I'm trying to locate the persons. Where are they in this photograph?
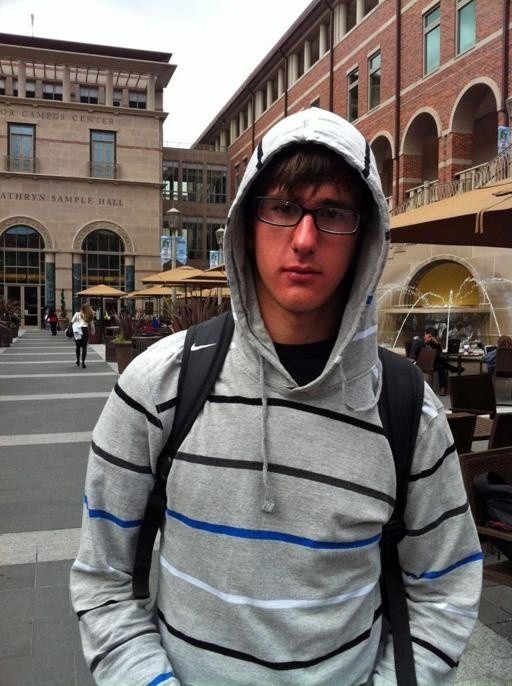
[71,304,96,368]
[46,309,59,336]
[69,106,483,686]
[482,336,512,374]
[410,326,464,396]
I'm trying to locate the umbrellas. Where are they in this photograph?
[76,263,230,318]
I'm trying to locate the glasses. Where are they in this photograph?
[248,192,365,235]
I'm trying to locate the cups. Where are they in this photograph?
[463,344,469,355]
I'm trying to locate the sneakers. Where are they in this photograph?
[75,361,80,367]
[439,387,447,397]
[81,362,88,368]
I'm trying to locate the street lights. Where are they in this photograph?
[209,223,224,316]
[160,207,188,335]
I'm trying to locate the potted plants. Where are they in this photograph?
[57,288,69,331]
[0,296,26,347]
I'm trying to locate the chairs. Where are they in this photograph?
[403,333,511,566]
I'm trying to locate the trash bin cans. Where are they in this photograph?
[131,337,162,360]
[107,328,120,361]
[58,319,69,331]
[89,322,102,344]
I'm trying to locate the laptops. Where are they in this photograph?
[442,338,460,354]
[485,345,497,356]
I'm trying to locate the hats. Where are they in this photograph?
[451,367,465,374]
